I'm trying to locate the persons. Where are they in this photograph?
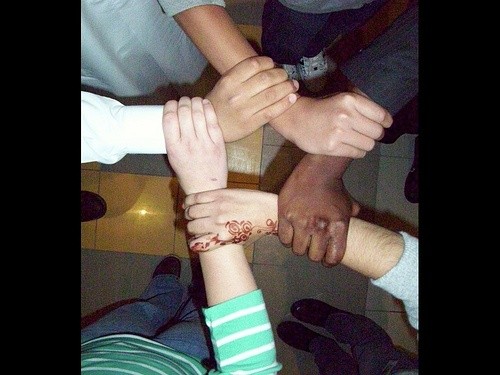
[181,187,418,375]
[279,1,418,267]
[81,55,299,167]
[81,95,285,375]
[81,0,394,159]
[81,189,107,223]
[260,0,390,98]
